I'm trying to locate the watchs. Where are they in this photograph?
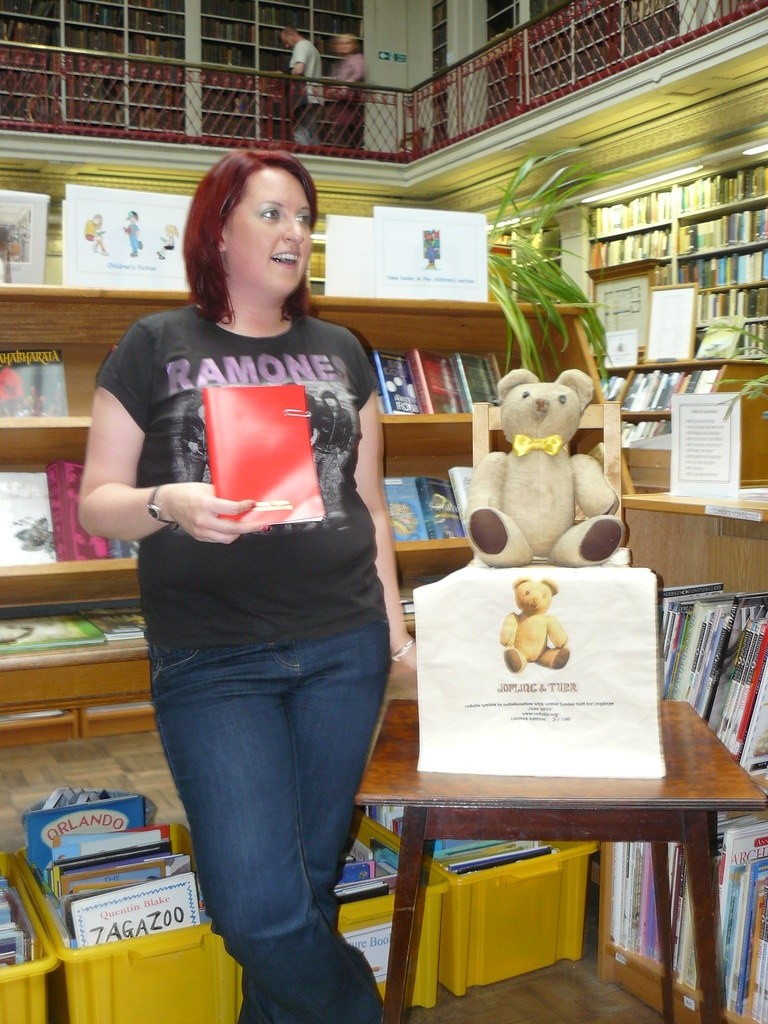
[147,485,176,524]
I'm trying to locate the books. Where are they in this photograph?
[0,0,678,149]
[0,163,768,1024]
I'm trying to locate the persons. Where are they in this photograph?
[79,149,415,1024]
[321,32,367,147]
[277,23,323,149]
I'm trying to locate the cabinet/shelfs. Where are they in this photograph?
[0,0,768,1024]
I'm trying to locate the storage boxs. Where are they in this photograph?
[14,821,238,1024]
[238,816,449,1017]
[0,851,62,1024]
[358,810,599,995]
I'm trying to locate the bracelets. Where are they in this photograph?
[391,638,414,663]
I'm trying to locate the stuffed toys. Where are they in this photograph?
[466,371,625,568]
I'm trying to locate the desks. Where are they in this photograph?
[354,699,768,1024]
